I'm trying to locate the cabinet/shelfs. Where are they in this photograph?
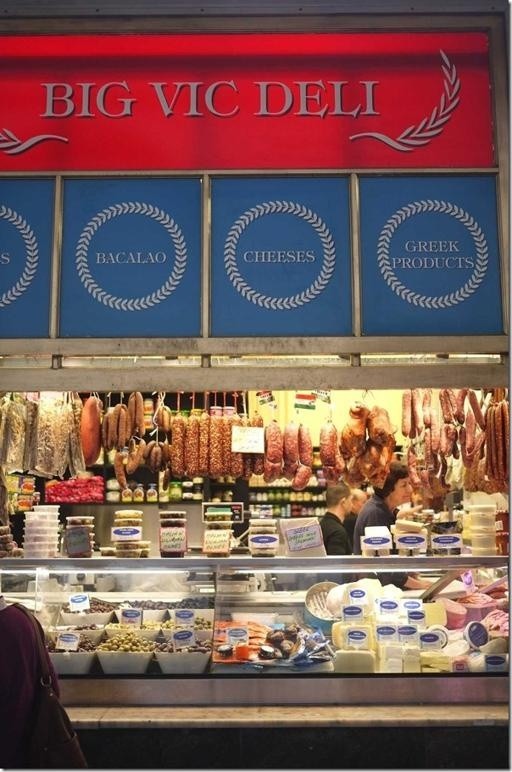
[237,445,402,546]
[64,393,244,501]
[212,557,509,706]
[1,558,211,707]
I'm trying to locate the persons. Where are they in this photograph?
[354,462,413,555]
[319,482,354,556]
[0,595,90,768]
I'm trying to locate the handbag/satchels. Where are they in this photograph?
[10,601,87,769]
